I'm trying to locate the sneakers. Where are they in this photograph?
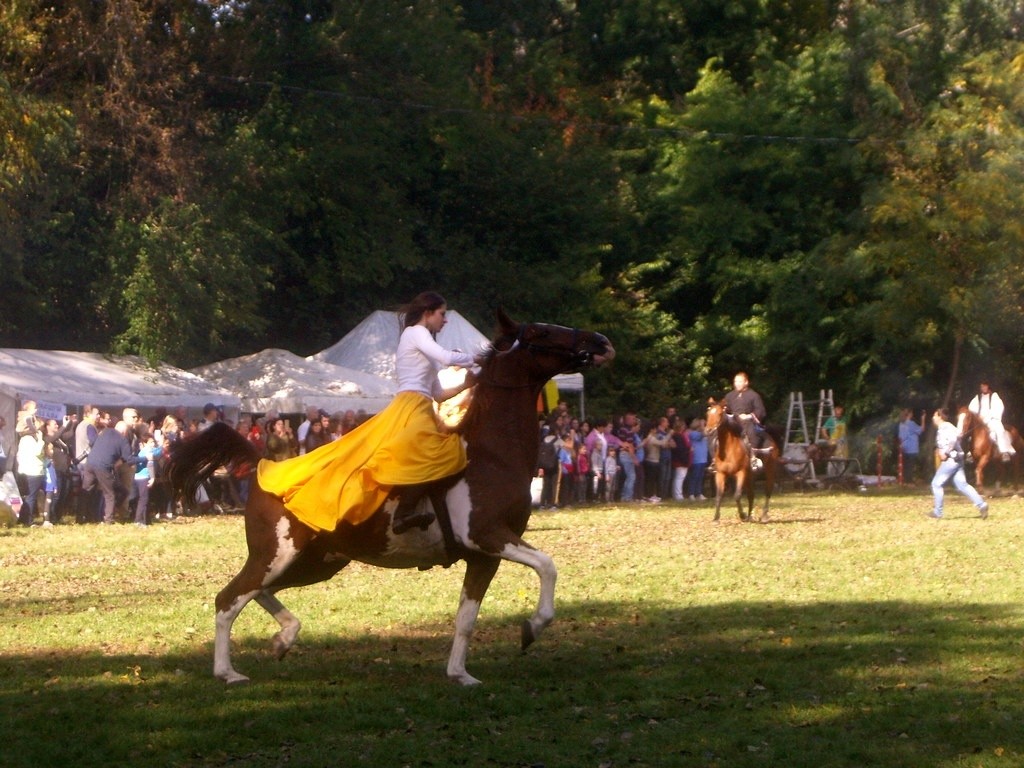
[980,504,989,522]
[926,510,943,519]
[392,502,436,535]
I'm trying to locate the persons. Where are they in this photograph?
[925,408,989,521]
[822,407,849,477]
[723,372,767,471]
[898,408,927,488]
[258,290,481,534]
[0,400,715,528]
[968,379,1016,462]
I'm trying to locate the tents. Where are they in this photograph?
[0,310,585,428]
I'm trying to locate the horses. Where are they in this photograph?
[147,308,617,688]
[954,404,1024,499]
[703,396,780,524]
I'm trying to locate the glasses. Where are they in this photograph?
[132,416,138,419]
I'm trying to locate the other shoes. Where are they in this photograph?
[648,495,662,503]
[698,494,707,500]
[689,495,696,500]
